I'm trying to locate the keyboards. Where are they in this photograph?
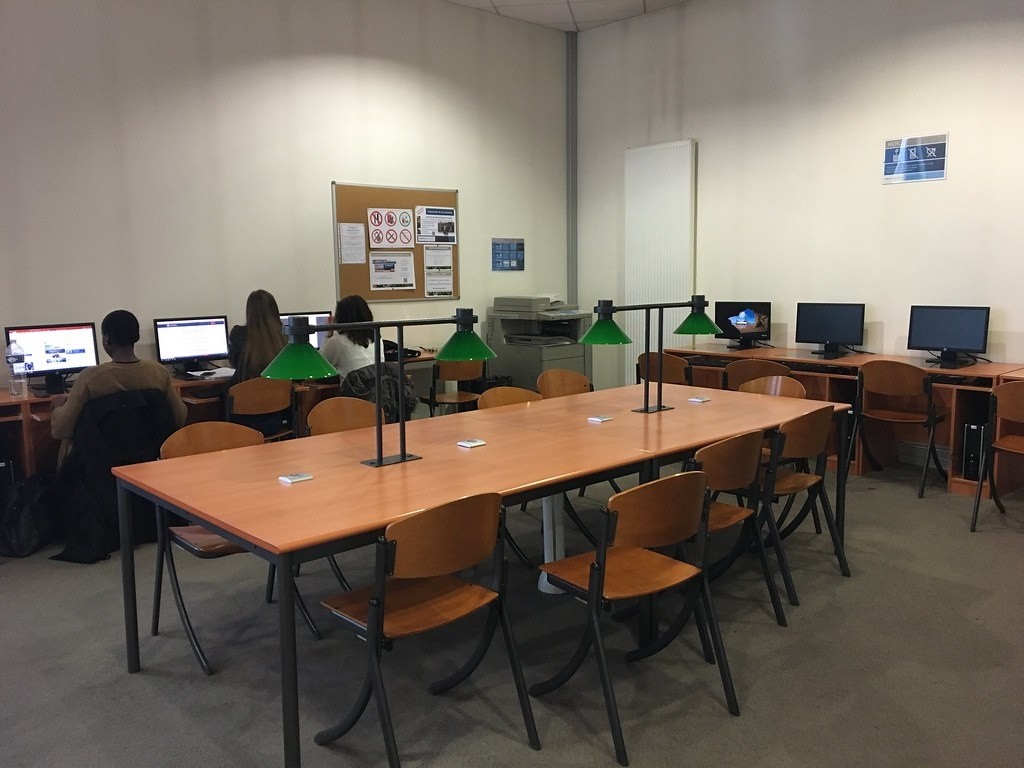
[192,388,226,399]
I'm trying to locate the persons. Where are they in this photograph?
[227,290,289,436]
[319,295,384,387]
[50,310,188,471]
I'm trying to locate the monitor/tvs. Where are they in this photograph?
[153,315,230,379]
[715,302,771,350]
[280,311,332,350]
[796,303,865,359]
[5,322,100,397]
[907,305,990,368]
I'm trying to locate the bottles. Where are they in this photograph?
[4,340,28,401]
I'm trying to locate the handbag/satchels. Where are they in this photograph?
[0,471,65,559]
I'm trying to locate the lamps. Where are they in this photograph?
[260,307,499,468]
[576,294,724,414]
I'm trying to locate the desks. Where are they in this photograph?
[0,350,459,477]
[454,382,853,646]
[662,343,1024,499]
[111,412,653,768]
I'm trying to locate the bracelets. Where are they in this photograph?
[327,335,332,338]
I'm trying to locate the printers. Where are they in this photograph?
[485,296,593,394]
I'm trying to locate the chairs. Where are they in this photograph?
[50,352,1024,768]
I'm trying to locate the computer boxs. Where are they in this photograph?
[0,457,15,510]
[839,397,856,462]
[962,423,992,483]
